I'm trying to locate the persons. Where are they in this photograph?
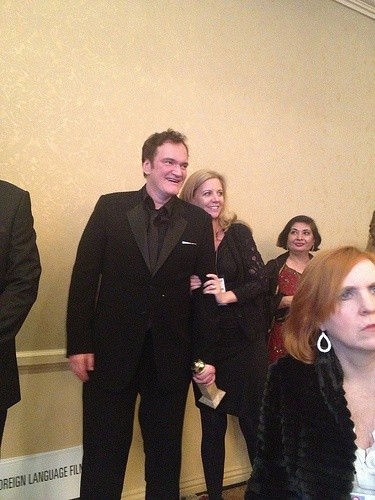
[243,246,375,500]
[66,129,219,500]
[178,171,271,500]
[265,215,323,367]
[0,181,42,445]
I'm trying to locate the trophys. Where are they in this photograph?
[190,358,226,410]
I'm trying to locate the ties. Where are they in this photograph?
[145,204,167,272]
